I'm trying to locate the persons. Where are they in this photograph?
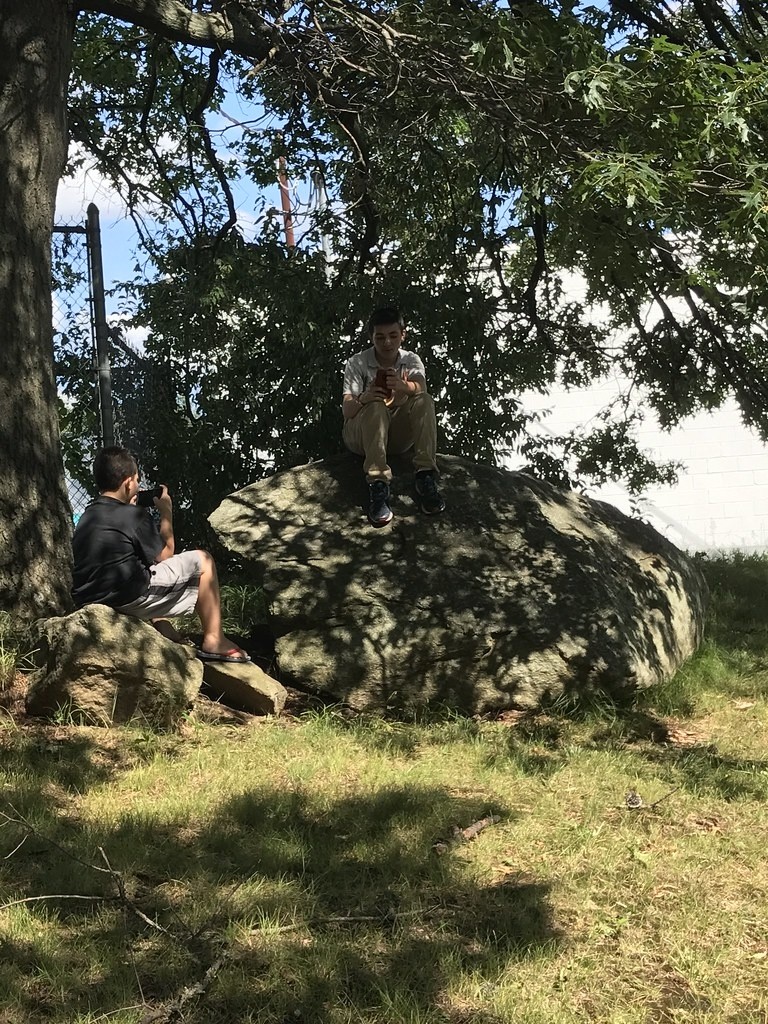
[73,447,252,662]
[342,307,445,524]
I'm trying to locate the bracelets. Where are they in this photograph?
[407,381,417,396]
[356,392,366,407]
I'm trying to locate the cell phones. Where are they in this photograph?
[137,488,163,508]
[375,370,392,399]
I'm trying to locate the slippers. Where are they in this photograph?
[176,636,198,647]
[197,647,249,663]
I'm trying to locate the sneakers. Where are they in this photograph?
[369,480,393,523]
[414,472,445,515]
